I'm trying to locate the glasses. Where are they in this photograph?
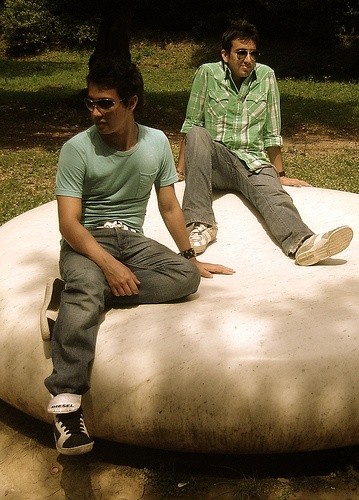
[230,50,260,59]
[84,97,125,112]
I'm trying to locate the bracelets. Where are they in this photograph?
[176,169,183,176]
[279,171,285,176]
[178,249,195,258]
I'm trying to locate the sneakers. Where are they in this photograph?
[294,225,354,266]
[47,393,94,455]
[188,224,218,254]
[40,276,66,342]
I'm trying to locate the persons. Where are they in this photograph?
[39,59,236,457]
[174,19,355,269]
